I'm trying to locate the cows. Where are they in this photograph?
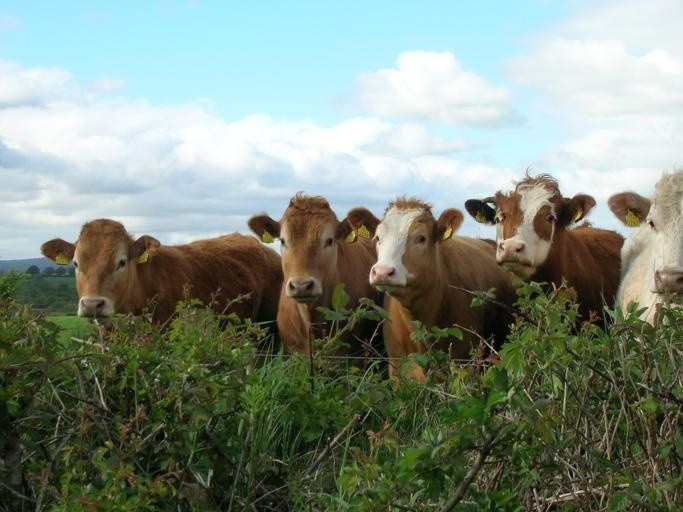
[607,167,682,349]
[464,166,626,341]
[40,219,283,358]
[247,191,384,378]
[368,195,530,396]
